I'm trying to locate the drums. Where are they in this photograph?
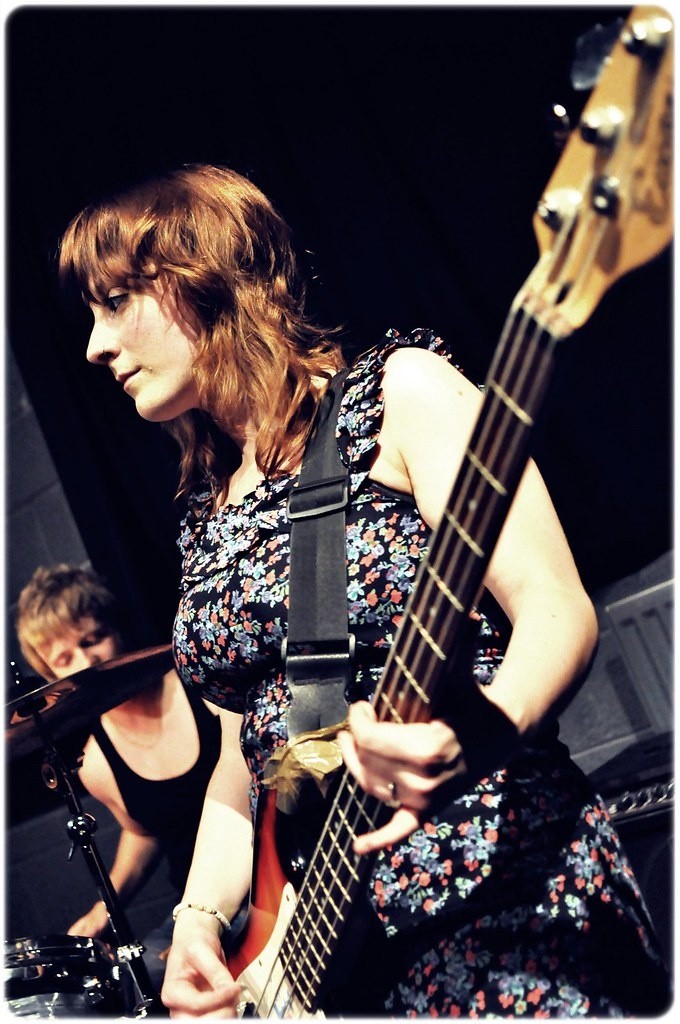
[5,929,146,1019]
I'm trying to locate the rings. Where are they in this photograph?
[385,782,400,808]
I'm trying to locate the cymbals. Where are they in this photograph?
[6,641,172,736]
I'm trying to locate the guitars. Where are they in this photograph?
[230,9,675,1019]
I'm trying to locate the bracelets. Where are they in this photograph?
[172,902,232,932]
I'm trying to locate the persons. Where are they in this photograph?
[17,563,221,938]
[58,163,675,1019]
